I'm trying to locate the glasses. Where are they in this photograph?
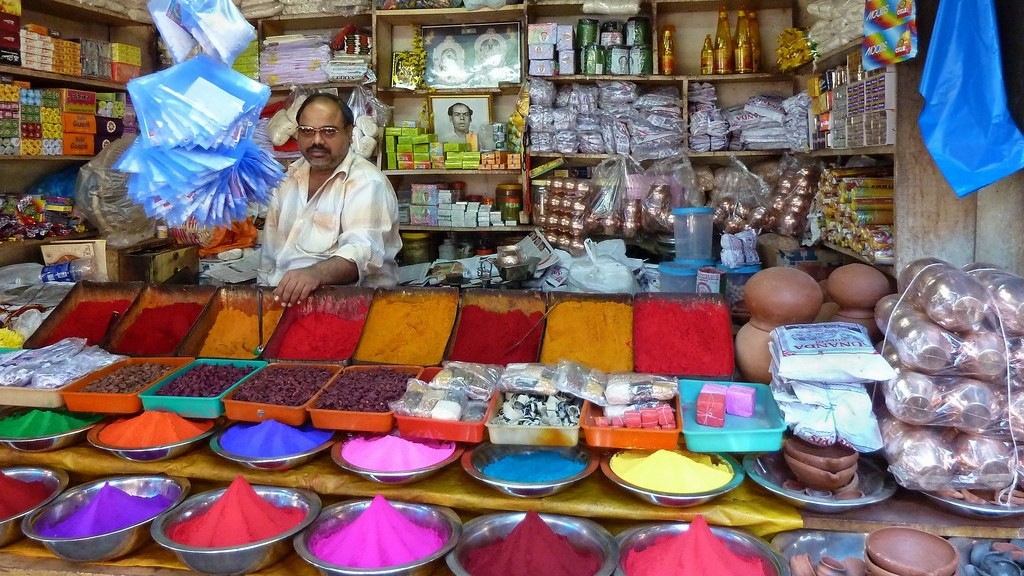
[297,126,347,140]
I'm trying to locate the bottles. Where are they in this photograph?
[401,229,493,265]
[15,258,98,286]
[658,208,760,309]
[661,28,674,77]
[700,9,760,76]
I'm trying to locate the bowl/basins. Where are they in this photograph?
[493,256,541,282]
[0,405,1024,576]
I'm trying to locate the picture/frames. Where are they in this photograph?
[426,93,493,142]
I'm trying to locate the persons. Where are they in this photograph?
[258,93,403,306]
[439,103,473,142]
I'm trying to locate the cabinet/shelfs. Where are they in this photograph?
[0,0,157,245]
[254,0,977,275]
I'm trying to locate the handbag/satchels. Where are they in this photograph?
[567,238,644,296]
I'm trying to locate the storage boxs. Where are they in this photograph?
[111,61,139,85]
[112,43,141,66]
[43,87,96,114]
[0,0,82,76]
[94,115,123,137]
[527,22,574,75]
[385,119,521,228]
[61,132,94,156]
[62,112,96,134]
[93,133,117,155]
